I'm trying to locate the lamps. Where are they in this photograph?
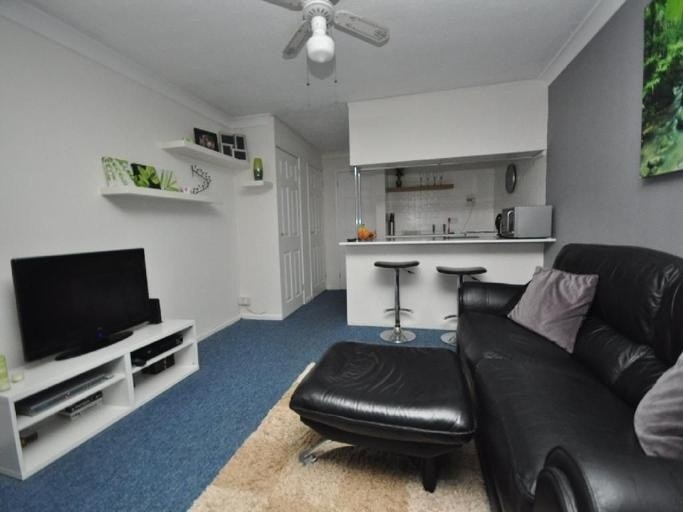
[305,15,335,63]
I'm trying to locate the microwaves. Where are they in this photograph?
[500,205,553,239]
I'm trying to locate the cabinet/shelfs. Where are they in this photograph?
[0,318,199,481]
[274,118,326,322]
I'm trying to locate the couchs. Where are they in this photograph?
[453,243,683,512]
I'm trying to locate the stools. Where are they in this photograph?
[288,339,476,493]
[436,265,486,348]
[375,261,419,344]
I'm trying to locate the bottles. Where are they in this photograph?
[254,157,263,180]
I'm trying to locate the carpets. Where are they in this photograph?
[184,361,491,511]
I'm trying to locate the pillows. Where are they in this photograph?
[506,265,598,356]
[632,349,683,459]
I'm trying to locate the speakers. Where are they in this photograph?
[149,298,161,324]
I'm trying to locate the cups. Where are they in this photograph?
[0,355,10,390]
[344,230,358,242]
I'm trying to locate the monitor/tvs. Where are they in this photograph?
[10,247,151,361]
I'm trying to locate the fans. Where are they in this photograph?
[264,0,388,59]
